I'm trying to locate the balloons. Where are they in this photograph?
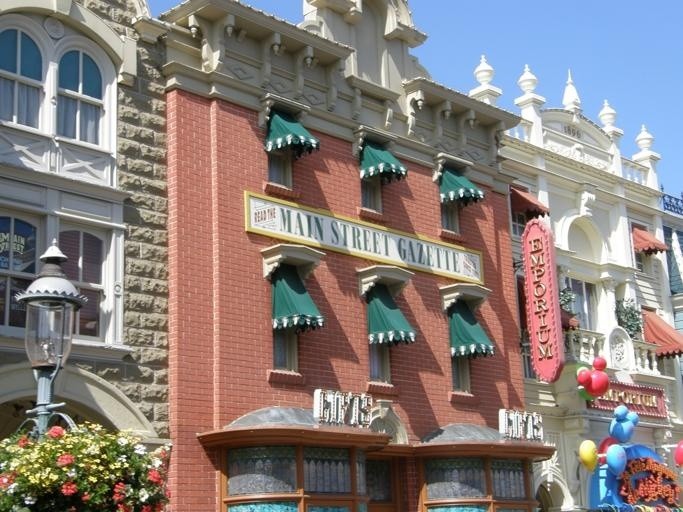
[608,444,627,480]
[674,441,683,465]
[615,405,628,421]
[579,440,597,470]
[574,356,609,401]
[627,412,640,426]
[608,419,634,442]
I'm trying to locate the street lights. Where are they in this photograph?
[15,237,89,442]
